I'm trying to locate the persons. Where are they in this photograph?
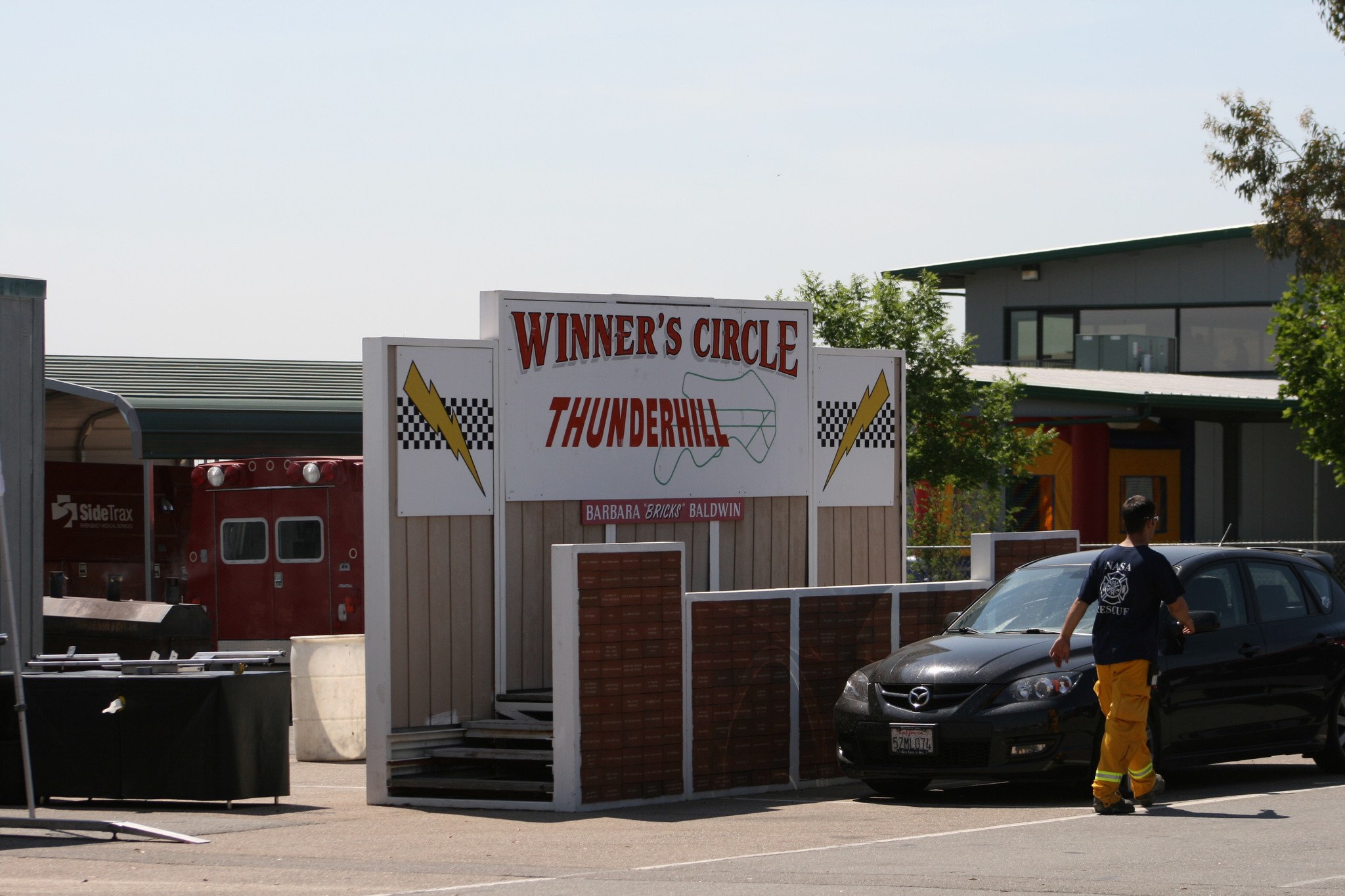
[1048,496,1196,814]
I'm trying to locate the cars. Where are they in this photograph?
[830,547,1345,804]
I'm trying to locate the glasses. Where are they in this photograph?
[1144,515,1159,523]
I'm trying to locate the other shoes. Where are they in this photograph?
[1136,773,1165,807]
[1093,795,1135,814]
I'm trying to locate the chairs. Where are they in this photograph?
[1186,575,1237,626]
[1256,583,1290,619]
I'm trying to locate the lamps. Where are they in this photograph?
[1020,265,1041,282]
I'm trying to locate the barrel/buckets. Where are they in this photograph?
[290,633,364,762]
